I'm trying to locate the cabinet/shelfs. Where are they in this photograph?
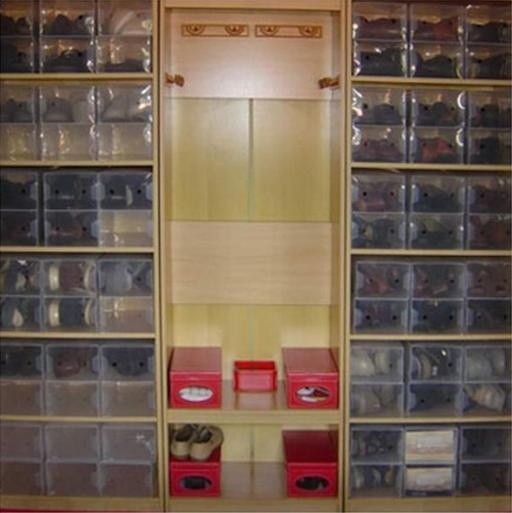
[0,2,161,513]
[162,2,346,512]
[349,1,511,506]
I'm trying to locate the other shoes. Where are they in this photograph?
[351,17,512,493]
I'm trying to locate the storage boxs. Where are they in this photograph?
[349,81,508,174]
[347,165,510,256]
[1,79,152,167]
[349,1,511,87]
[349,421,511,500]
[282,345,341,411]
[3,2,152,81]
[0,250,154,340]
[233,359,277,392]
[2,167,153,253]
[1,419,155,498]
[283,429,338,497]
[349,338,511,420]
[3,336,153,421]
[168,441,222,498]
[167,346,224,409]
[349,255,509,341]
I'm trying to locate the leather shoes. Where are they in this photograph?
[170,424,223,461]
[0,9,152,123]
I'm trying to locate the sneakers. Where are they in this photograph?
[0,174,151,378]
